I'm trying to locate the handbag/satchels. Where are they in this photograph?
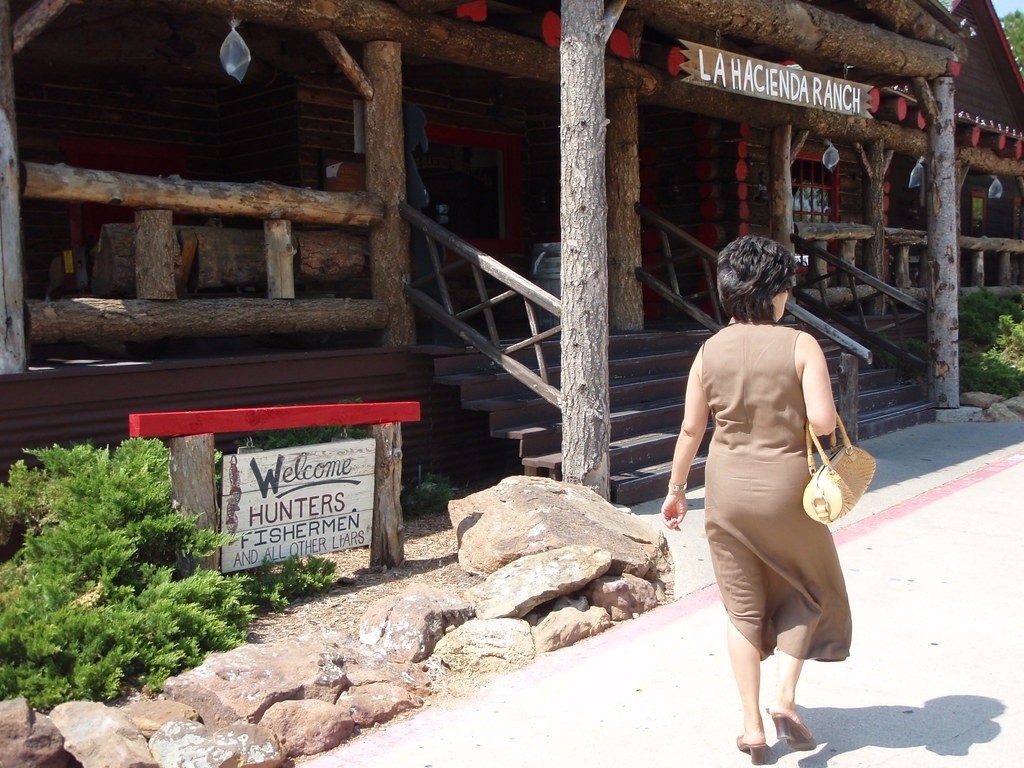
[802,412,876,524]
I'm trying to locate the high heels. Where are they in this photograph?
[771,712,819,752]
[736,734,768,765]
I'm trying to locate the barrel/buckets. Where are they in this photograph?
[529,242,560,340]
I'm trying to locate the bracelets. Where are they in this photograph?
[667,483,687,491]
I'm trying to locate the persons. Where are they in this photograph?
[661,236,853,766]
